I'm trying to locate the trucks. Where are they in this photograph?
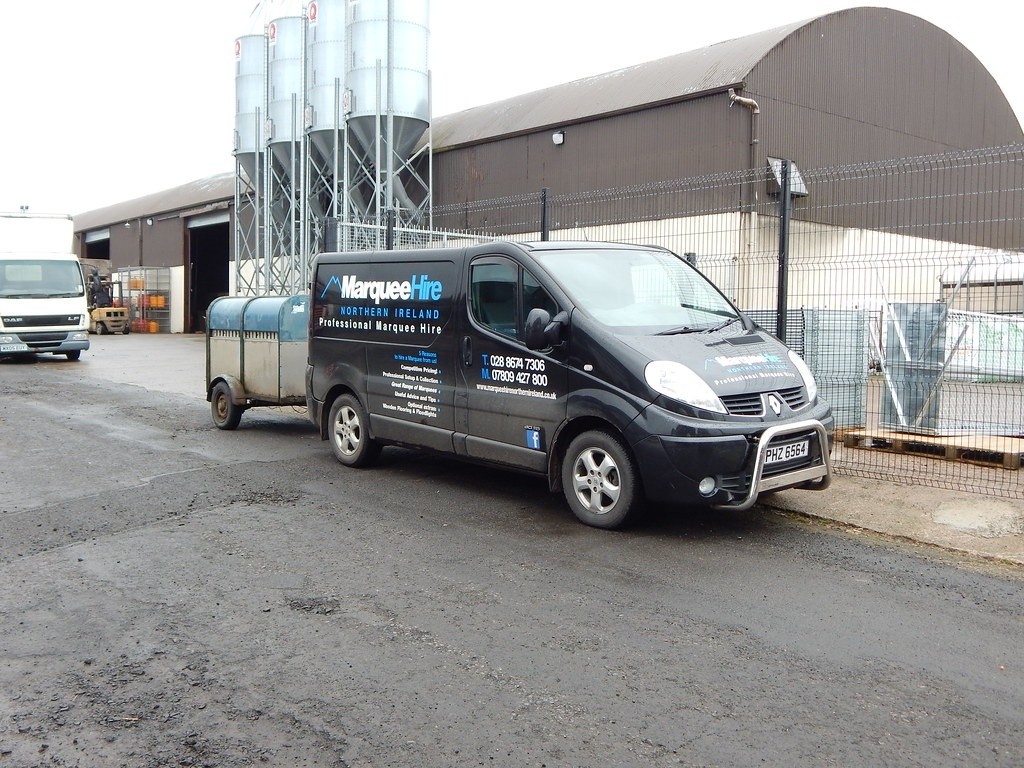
[0,212,90,363]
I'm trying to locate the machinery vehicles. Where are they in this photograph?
[82,274,130,335]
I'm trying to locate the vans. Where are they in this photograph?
[301,240,835,533]
